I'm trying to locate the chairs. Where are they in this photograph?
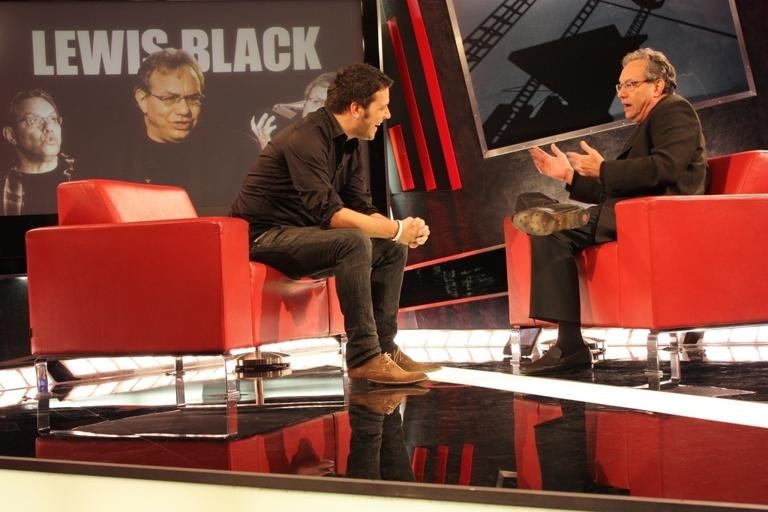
[502,149,767,391]
[24,179,346,442]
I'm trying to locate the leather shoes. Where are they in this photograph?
[386,347,441,373]
[347,351,429,386]
[518,339,595,376]
[510,203,591,237]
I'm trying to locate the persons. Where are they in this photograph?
[228,62,443,386]
[510,49,710,376]
[0,86,77,215]
[250,68,336,151]
[533,365,630,495]
[87,48,260,208]
[287,376,430,481]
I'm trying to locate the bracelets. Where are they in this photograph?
[390,219,402,242]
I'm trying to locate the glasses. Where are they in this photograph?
[144,88,206,107]
[615,77,660,94]
[306,95,325,110]
[14,113,64,129]
